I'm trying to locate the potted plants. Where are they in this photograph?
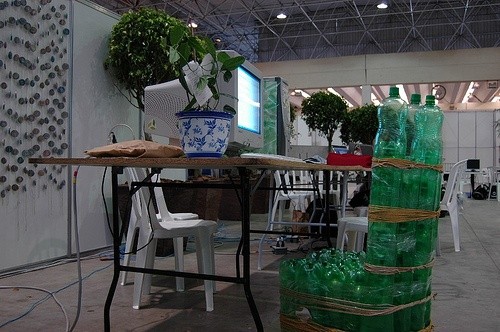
[299,89,350,205]
[156,19,246,156]
[104,9,225,257]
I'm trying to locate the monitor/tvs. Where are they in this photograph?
[144,50,264,156]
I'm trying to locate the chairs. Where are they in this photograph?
[479,167,500,203]
[337,216,372,254]
[280,88,443,332]
[132,167,218,312]
[120,165,198,285]
[434,158,468,255]
[269,156,345,233]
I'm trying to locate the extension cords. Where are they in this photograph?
[272,246,287,255]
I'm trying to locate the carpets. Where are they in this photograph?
[0,209,317,332]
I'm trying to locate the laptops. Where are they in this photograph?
[332,145,348,155]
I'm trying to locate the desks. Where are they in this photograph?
[30,156,372,332]
[444,169,479,198]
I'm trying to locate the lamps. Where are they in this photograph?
[277,9,286,19]
[376,0,388,9]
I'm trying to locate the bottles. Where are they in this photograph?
[410,95,444,166]
[404,94,422,161]
[361,161,441,332]
[373,87,409,159]
[278,248,366,332]
